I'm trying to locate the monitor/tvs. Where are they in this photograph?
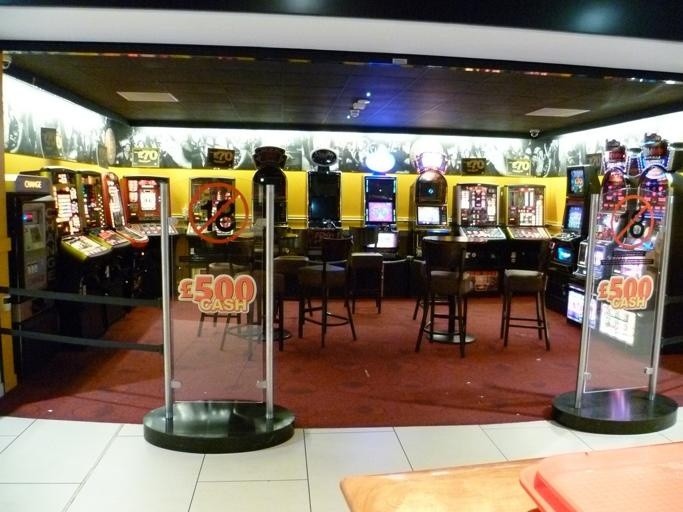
[255,201,287,225]
[459,183,499,226]
[308,196,340,221]
[419,207,441,226]
[554,168,662,278]
[501,185,546,225]
[366,202,394,223]
[47,168,170,234]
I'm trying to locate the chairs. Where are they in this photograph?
[498,239,553,352]
[301,238,357,348]
[413,258,468,335]
[275,235,314,316]
[416,239,476,357]
[343,225,384,314]
[221,241,285,353]
[196,230,254,338]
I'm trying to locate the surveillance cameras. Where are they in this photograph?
[1,54,14,70]
[530,130,541,137]
